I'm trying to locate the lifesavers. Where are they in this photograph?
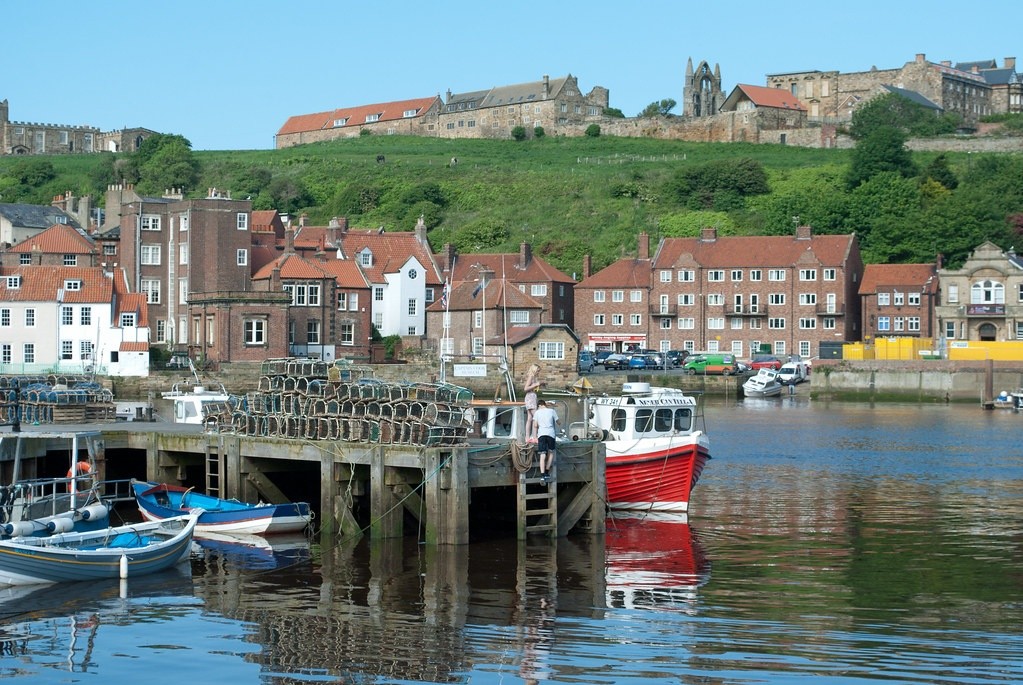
[66,461,98,497]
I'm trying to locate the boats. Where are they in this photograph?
[130,478,313,537]
[1,472,112,540]
[985,389,1023,411]
[440,252,546,444]
[567,376,712,512]
[0,511,201,581]
[741,367,783,396]
[108,381,235,425]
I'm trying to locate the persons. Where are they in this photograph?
[532,400,561,479]
[523,364,544,445]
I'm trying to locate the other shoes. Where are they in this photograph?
[525,438,538,444]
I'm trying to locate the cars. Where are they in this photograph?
[579,348,812,386]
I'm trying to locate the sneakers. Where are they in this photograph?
[545,474,551,479]
[540,476,546,486]
